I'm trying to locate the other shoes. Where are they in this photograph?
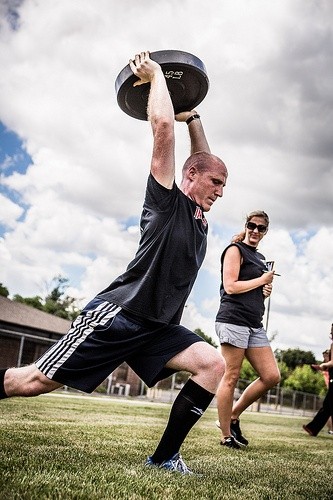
[328,429,333,434]
[303,424,313,437]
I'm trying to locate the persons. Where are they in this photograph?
[0,49,227,478]
[321,350,333,433]
[302,323,333,436]
[215,210,280,449]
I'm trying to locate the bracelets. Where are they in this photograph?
[186,115,200,125]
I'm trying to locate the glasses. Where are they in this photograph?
[246,222,267,233]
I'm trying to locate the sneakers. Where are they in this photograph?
[220,435,247,450]
[216,418,249,447]
[145,453,193,474]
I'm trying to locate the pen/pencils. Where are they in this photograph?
[263,270,282,277]
